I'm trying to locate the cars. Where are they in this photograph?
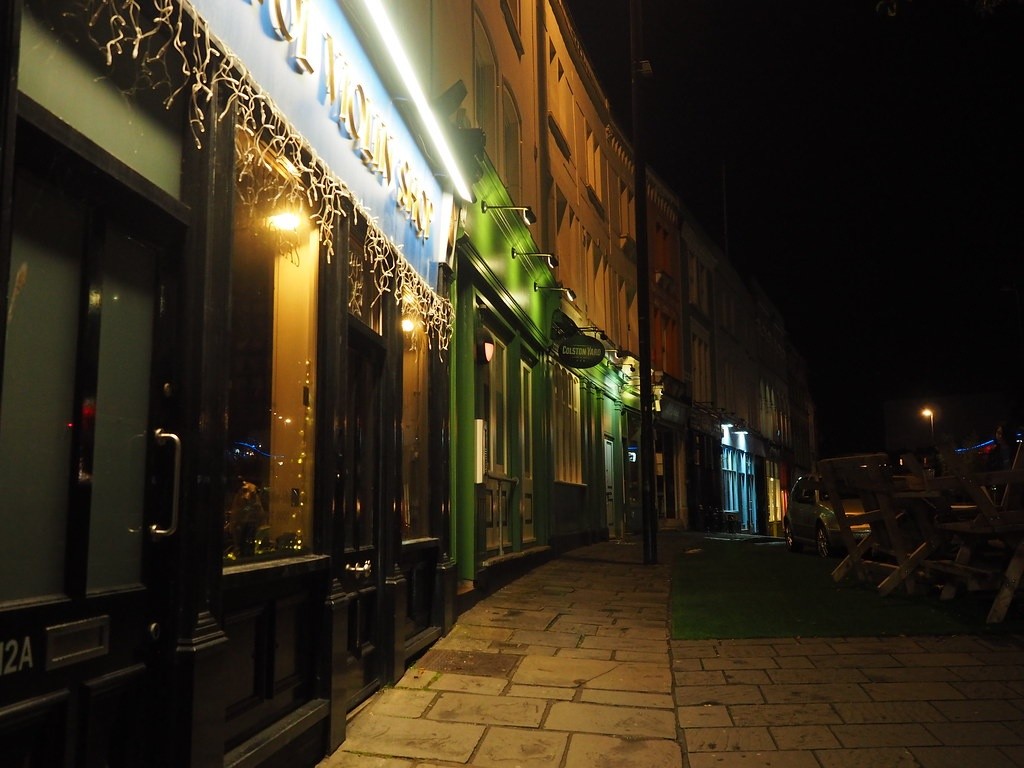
[783,474,888,558]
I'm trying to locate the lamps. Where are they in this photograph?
[400,311,415,331]
[610,363,635,372]
[695,400,746,428]
[582,329,608,340]
[534,281,577,302]
[481,199,537,225]
[605,349,623,359]
[482,329,494,364]
[512,247,559,269]
[256,165,303,232]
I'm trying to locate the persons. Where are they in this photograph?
[985,420,1017,494]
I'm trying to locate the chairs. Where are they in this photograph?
[816,440,1024,626]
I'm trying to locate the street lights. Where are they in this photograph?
[920,406,934,443]
